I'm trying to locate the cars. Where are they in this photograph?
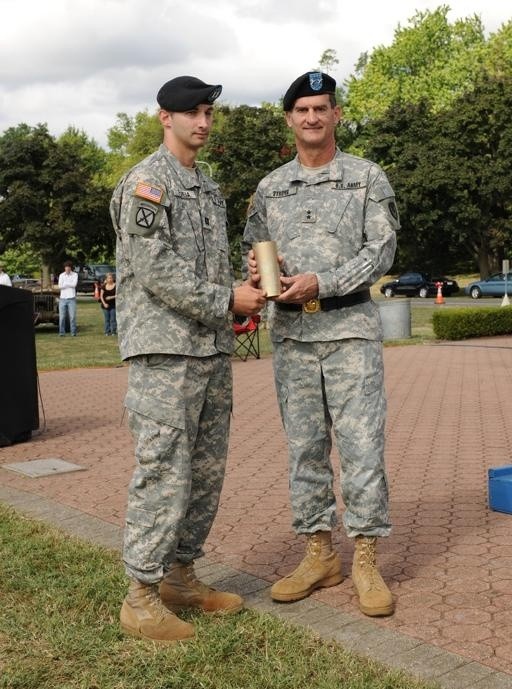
[379,269,463,298]
[14,264,118,295]
[465,271,511,299]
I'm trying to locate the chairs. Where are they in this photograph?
[232,315,261,362]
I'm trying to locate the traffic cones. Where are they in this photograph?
[433,285,446,305]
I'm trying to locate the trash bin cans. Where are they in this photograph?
[0,285,40,448]
[379,301,412,340]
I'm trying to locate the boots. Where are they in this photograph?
[270,531,344,601]
[119,583,196,645]
[160,558,245,617]
[351,535,394,616]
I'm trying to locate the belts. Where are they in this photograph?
[277,290,371,312]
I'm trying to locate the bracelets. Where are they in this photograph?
[229,288,235,311]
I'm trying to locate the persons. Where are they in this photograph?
[100,273,116,337]
[108,75,271,632]
[240,70,401,616]
[58,261,79,336]
[1,266,12,287]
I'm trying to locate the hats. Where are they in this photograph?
[283,71,336,111]
[157,76,222,111]
